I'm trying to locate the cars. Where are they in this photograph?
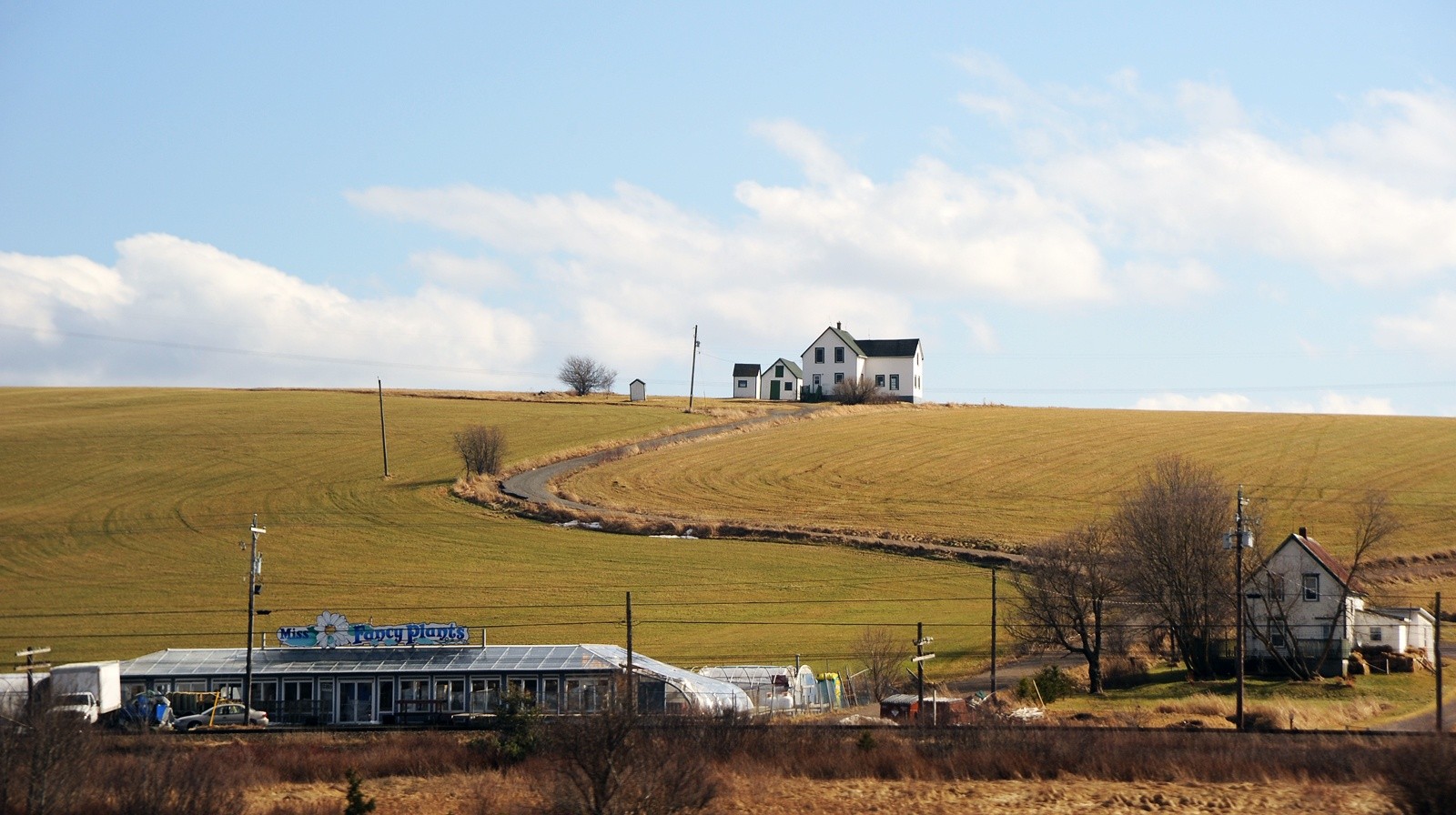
[171,702,271,733]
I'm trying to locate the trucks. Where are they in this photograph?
[43,660,122,725]
